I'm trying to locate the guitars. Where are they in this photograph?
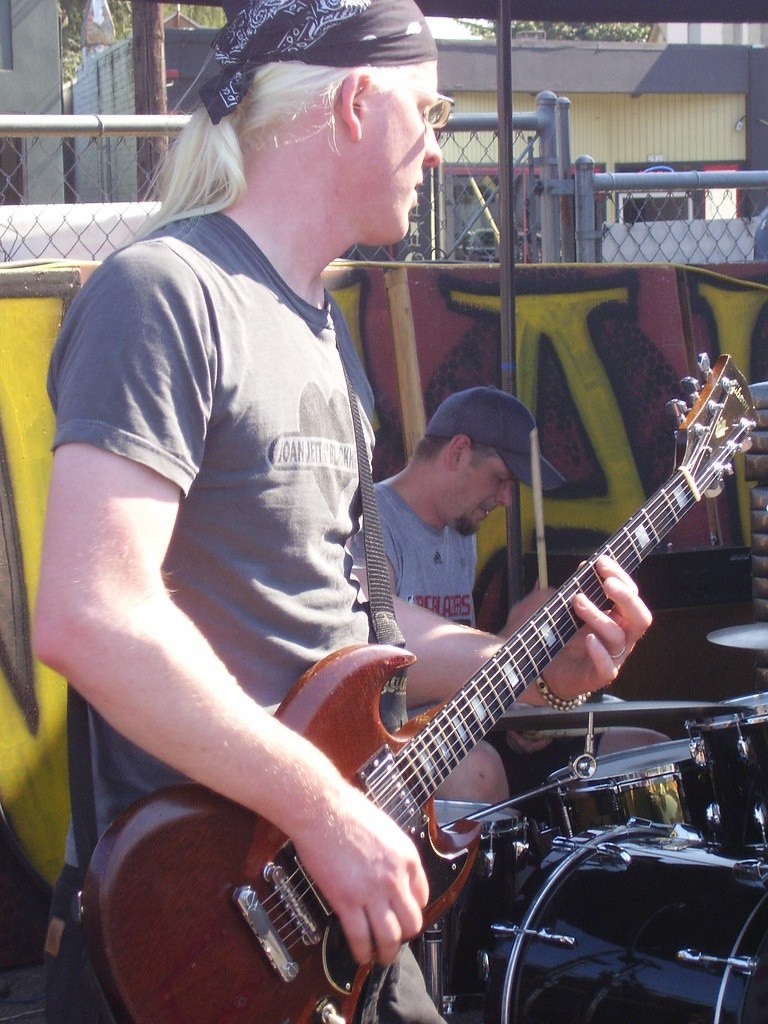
[77,351,763,1024]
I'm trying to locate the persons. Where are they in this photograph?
[350,387,676,830]
[31,0,653,1024]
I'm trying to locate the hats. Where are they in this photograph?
[424,384,567,490]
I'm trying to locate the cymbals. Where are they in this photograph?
[503,698,749,727]
[704,621,767,652]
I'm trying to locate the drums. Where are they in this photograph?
[462,819,768,1024]
[546,735,693,824]
[689,692,768,854]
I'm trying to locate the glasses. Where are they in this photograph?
[335,71,455,129]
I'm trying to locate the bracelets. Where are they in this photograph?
[535,674,592,712]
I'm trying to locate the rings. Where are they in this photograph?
[610,646,625,658]
